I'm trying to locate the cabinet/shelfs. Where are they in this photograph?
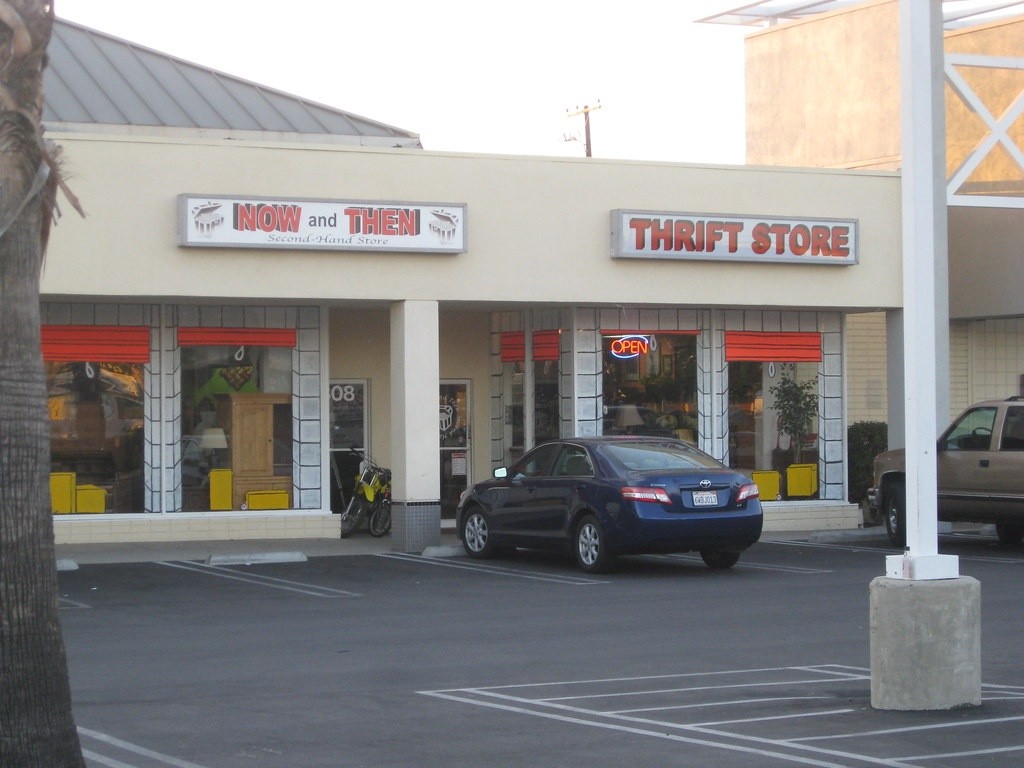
[215,392,292,508]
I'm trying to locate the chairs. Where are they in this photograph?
[566,456,591,475]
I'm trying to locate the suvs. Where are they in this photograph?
[873,396,1024,548]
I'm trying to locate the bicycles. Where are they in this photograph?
[334,447,394,538]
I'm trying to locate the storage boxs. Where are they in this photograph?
[752,469,779,501]
[786,463,817,497]
[206,470,234,511]
[48,470,76,514]
[245,489,292,510]
[74,484,106,513]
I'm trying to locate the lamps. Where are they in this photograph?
[199,428,228,467]
[612,404,646,436]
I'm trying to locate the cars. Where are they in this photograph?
[456,435,764,577]
